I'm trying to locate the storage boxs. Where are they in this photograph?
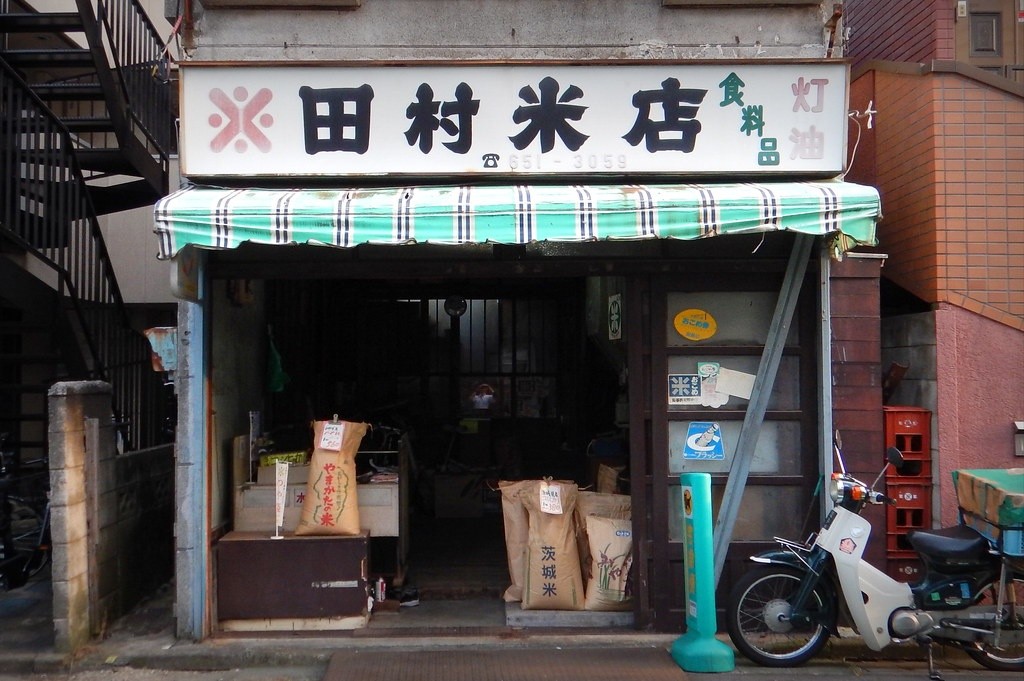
[423,469,483,519]
[883,405,932,482]
[888,558,926,585]
[886,481,933,558]
[952,468,1024,556]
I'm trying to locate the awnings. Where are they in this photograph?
[154,184,883,261]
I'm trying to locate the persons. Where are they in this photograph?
[470,384,497,409]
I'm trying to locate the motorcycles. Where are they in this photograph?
[725,427,1023,681]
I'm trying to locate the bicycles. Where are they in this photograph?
[0,456,52,590]
